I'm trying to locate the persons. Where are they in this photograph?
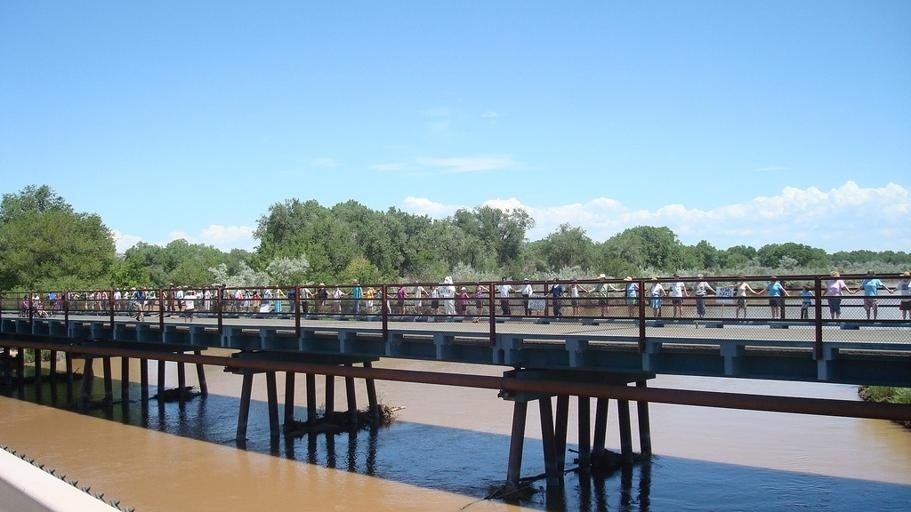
[823,270,853,319]
[162,281,218,317]
[757,275,790,319]
[799,285,815,319]
[113,285,156,322]
[547,274,716,317]
[395,272,534,315]
[728,281,757,318]
[892,272,911,320]
[222,283,295,312]
[23,290,108,317]
[853,269,892,319]
[299,279,393,313]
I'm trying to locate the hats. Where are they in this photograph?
[524,278,529,281]
[673,272,679,277]
[624,276,632,281]
[597,274,606,278]
